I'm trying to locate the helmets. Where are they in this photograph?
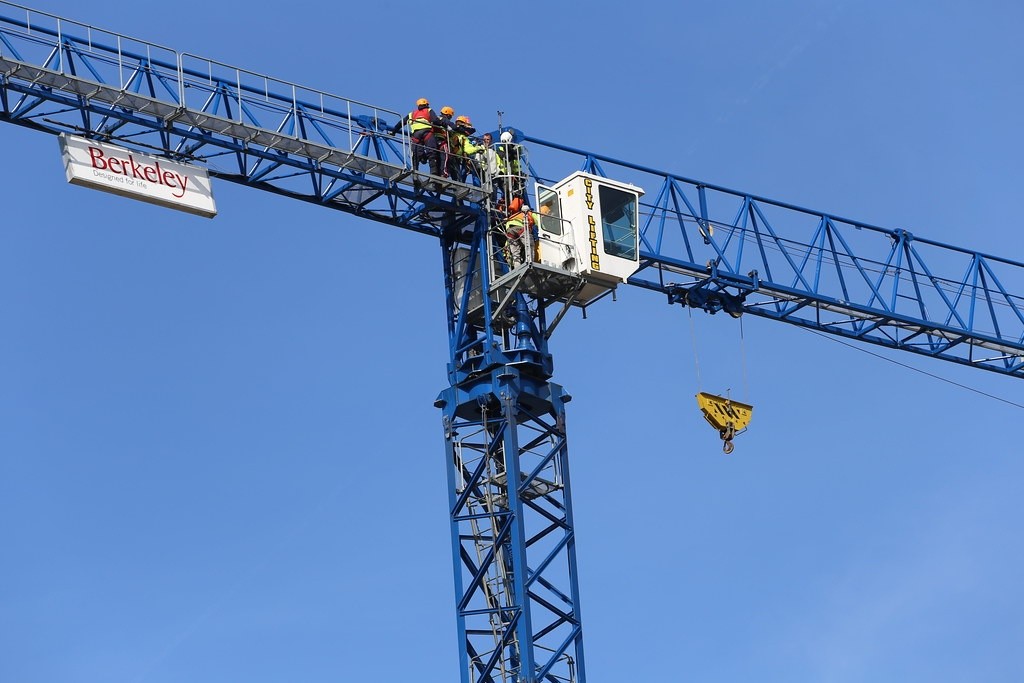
[500,132,512,142]
[541,206,550,217]
[456,116,467,124]
[417,99,429,106]
[440,106,453,117]
[521,205,530,212]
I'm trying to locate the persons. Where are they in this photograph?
[385,98,551,269]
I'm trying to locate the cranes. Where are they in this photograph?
[0,2,1023,682]
